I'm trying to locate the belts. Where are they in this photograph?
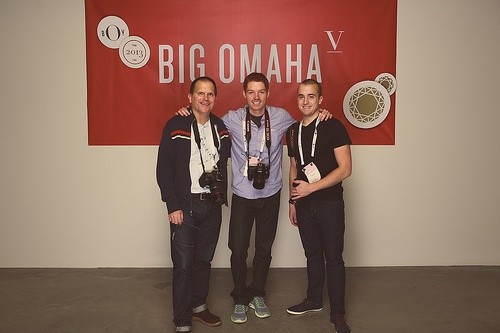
[191,192,211,200]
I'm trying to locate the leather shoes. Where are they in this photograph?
[193,308,221,326]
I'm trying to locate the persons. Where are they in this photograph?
[176,72,332,323]
[157,77,232,333]
[286,79,352,333]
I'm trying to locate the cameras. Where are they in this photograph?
[248,163,269,190]
[288,178,311,207]
[199,169,226,206]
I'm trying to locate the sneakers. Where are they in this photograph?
[331,316,350,333]
[249,296,270,318]
[287,298,322,314]
[231,305,248,323]
